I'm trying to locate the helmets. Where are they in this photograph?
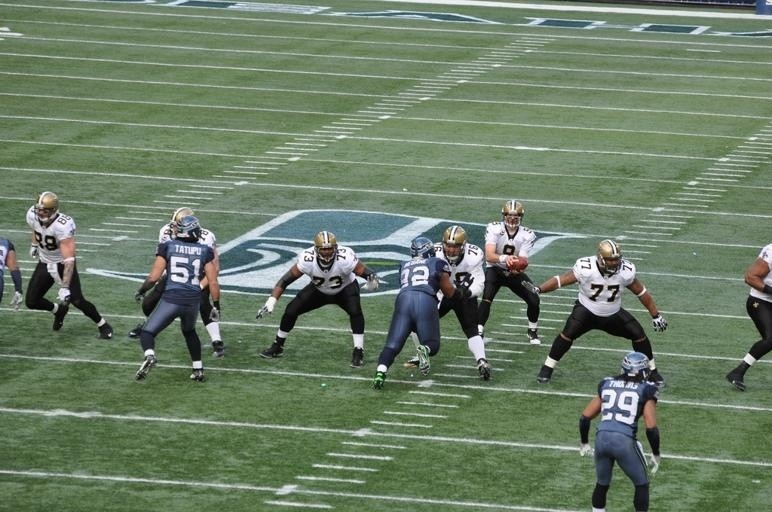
[501,200,525,228]
[169,207,194,232]
[620,352,650,378]
[441,225,468,261]
[410,237,436,260]
[596,239,623,275]
[314,231,337,262]
[173,215,202,240]
[31,191,59,223]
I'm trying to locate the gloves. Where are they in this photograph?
[521,281,541,295]
[579,442,595,457]
[649,453,661,473]
[29,243,39,259]
[10,289,23,311]
[57,287,71,306]
[652,312,668,332]
[256,297,277,319]
[209,300,220,322]
[360,274,379,292]
[134,289,146,304]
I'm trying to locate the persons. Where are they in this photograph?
[525,239,668,386]
[26,192,112,340]
[372,237,456,389]
[478,200,540,345]
[404,225,491,382]
[134,217,220,385]
[726,242,772,390]
[0,238,23,309]
[256,231,379,367]
[580,353,661,511]
[128,207,224,359]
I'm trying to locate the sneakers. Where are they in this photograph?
[536,365,553,385]
[407,356,420,366]
[416,345,431,377]
[368,371,386,390]
[648,367,665,388]
[189,368,205,381]
[53,304,69,331]
[725,368,746,392]
[349,345,364,368]
[526,328,540,345]
[98,323,113,339]
[135,354,158,380]
[477,358,491,380]
[127,321,146,337]
[212,340,225,358]
[259,342,283,359]
